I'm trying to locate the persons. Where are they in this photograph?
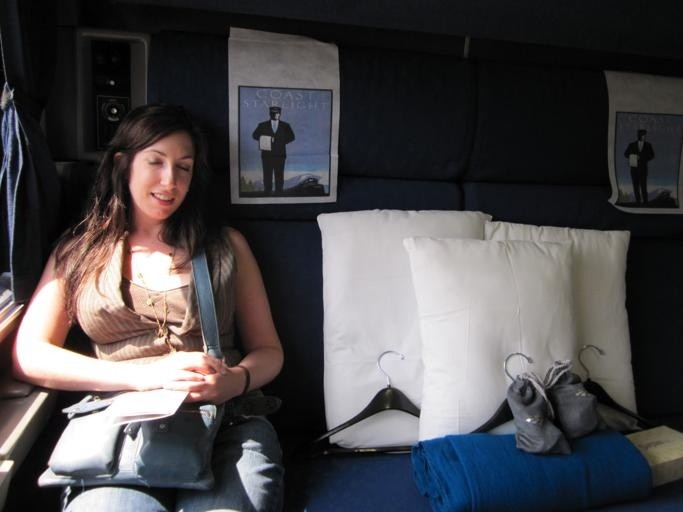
[11,100,286,512]
[624,128,655,202]
[252,105,296,190]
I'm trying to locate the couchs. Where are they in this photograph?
[41,10,683,512]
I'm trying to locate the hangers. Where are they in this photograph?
[470,353,540,436]
[320,351,420,441]
[580,345,639,427]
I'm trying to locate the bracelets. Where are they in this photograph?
[236,362,252,396]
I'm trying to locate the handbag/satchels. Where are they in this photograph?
[37,390,224,492]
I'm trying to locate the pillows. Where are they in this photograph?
[483,224,636,416]
[318,210,490,445]
[405,237,579,441]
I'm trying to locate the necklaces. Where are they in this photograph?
[126,231,179,352]
